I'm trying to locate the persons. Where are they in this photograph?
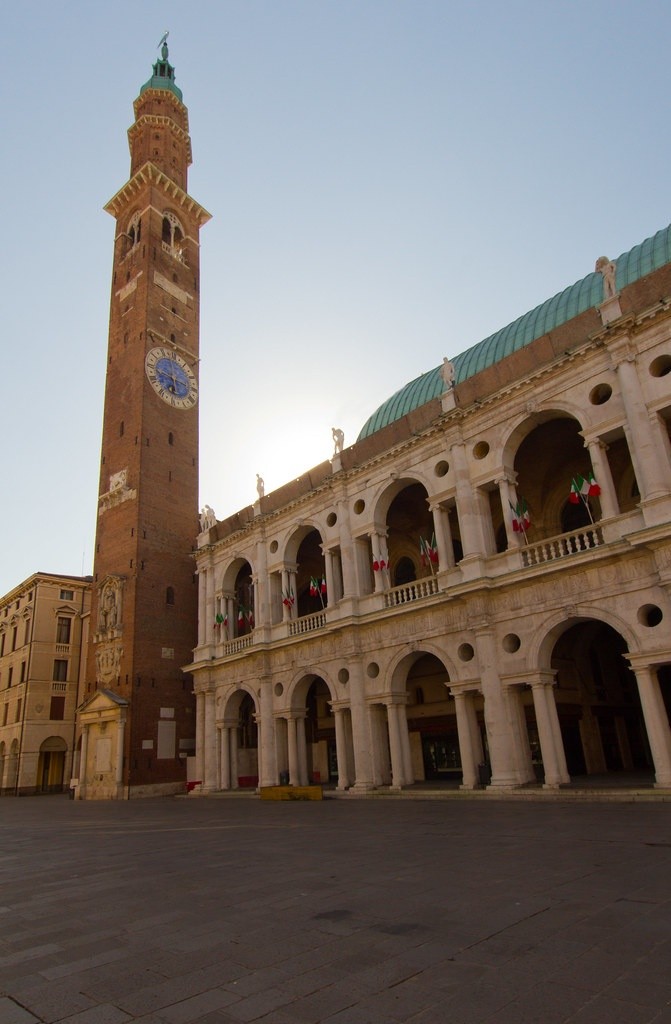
[595,256,616,300]
[256,474,264,498]
[198,504,216,532]
[332,428,344,455]
[440,357,455,389]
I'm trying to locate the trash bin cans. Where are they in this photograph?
[280,771,289,786]
[313,771,321,784]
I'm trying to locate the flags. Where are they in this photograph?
[587,471,601,497]
[372,550,391,572]
[509,502,523,533]
[310,574,326,597]
[420,532,439,567]
[576,473,590,495]
[515,501,522,525]
[281,586,294,610]
[568,478,581,504]
[213,613,228,630]
[237,604,255,630]
[521,496,531,529]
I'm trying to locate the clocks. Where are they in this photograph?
[144,346,198,411]
